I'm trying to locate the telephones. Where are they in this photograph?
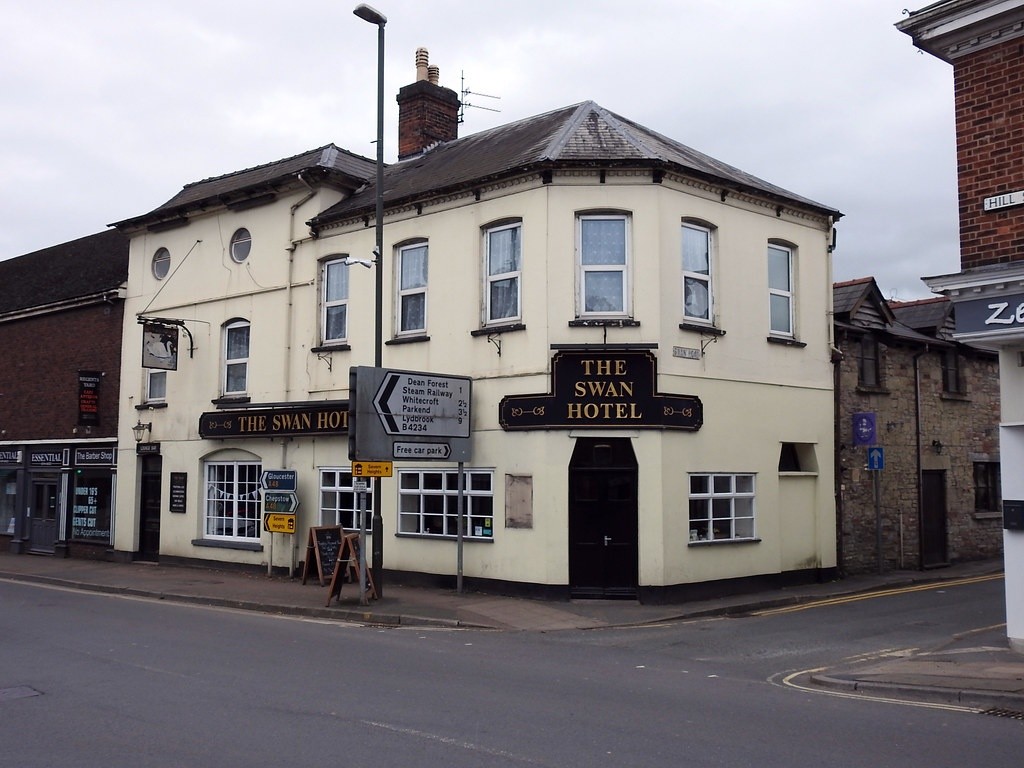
[312,525,344,578]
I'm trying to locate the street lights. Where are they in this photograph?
[352,2,387,600]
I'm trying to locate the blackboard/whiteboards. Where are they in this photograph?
[347,534,375,595]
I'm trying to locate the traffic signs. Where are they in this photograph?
[349,364,474,464]
[262,513,298,534]
[264,491,300,513]
[259,469,298,493]
